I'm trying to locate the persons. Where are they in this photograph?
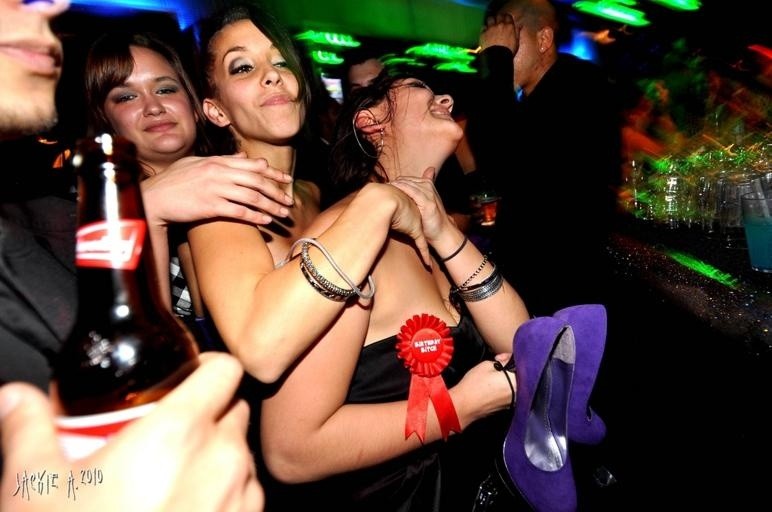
[0,1,626,512]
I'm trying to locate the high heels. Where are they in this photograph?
[503,304,607,510]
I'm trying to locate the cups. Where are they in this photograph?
[743,190,772,273]
[471,192,502,227]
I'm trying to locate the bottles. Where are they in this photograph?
[47,134,200,465]
